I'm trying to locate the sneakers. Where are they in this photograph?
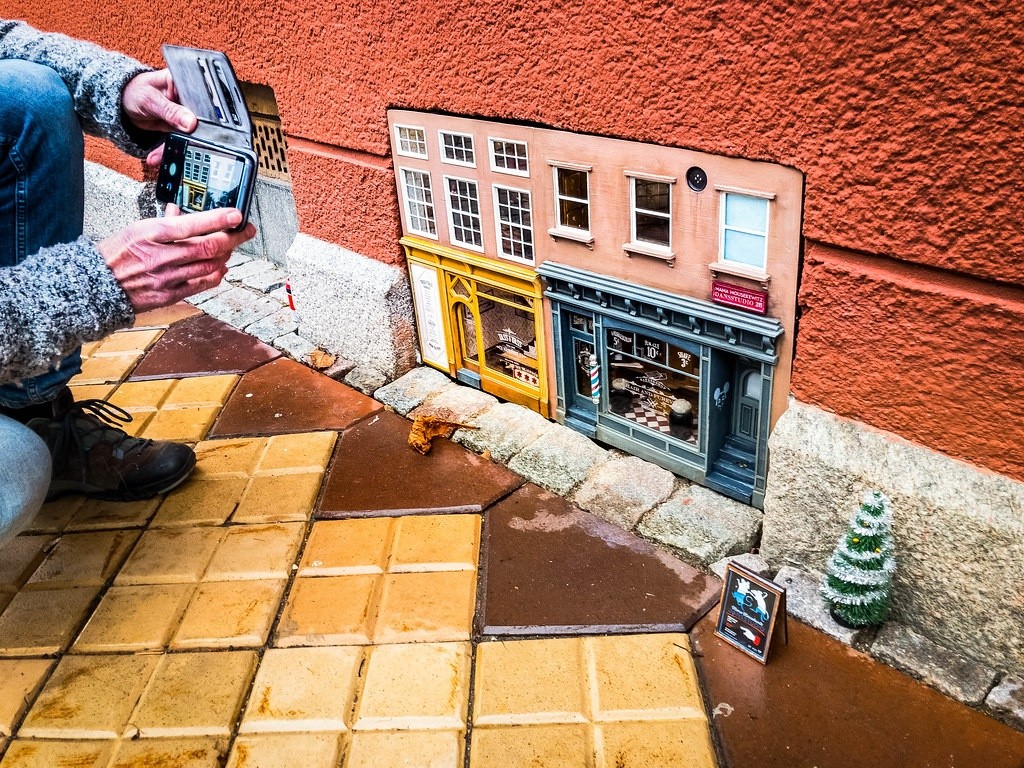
[23,386,197,503]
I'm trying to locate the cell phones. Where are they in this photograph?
[155,133,255,231]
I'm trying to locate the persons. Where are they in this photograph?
[0,17,257,542]
